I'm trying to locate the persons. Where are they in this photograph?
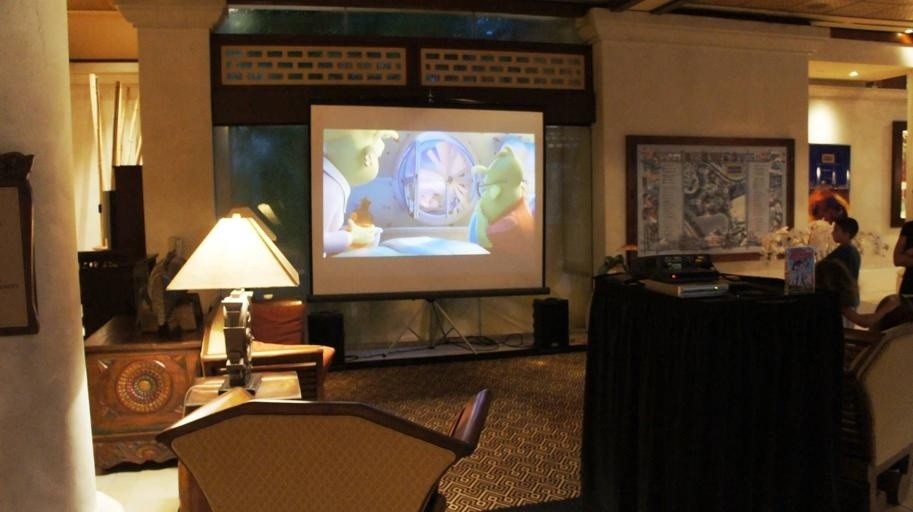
[891,221,912,298]
[816,216,862,329]
[147,253,188,341]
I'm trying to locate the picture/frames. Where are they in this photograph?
[889,121,907,226]
[621,132,798,267]
[0,151,43,336]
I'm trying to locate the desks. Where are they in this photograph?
[184,371,304,413]
[574,270,844,511]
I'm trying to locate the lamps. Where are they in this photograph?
[164,213,301,396]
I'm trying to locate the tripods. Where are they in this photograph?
[381,299,478,357]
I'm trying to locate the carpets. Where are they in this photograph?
[313,349,592,508]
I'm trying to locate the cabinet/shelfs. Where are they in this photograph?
[83,309,206,477]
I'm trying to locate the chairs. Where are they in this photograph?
[843,319,911,506]
[154,385,492,510]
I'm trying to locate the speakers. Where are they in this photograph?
[308,311,345,363]
[533,299,570,344]
